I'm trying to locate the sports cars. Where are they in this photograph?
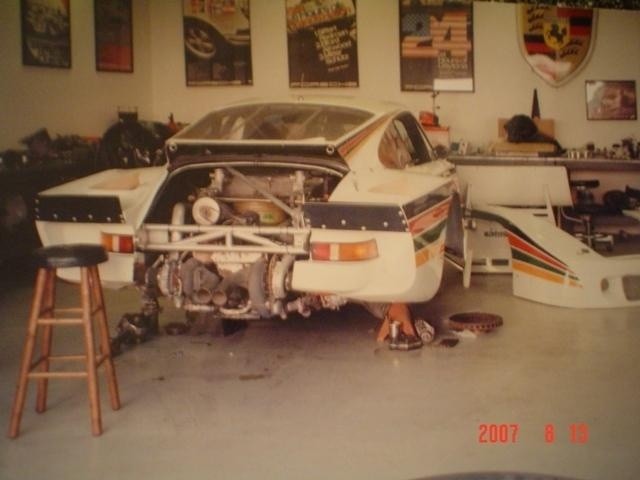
[29,80,480,324]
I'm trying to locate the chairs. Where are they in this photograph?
[564,177,617,255]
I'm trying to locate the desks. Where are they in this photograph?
[448,150,640,206]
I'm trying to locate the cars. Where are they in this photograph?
[184,2,251,62]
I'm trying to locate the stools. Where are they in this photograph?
[4,240,127,441]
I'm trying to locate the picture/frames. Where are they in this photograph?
[583,76,638,125]
